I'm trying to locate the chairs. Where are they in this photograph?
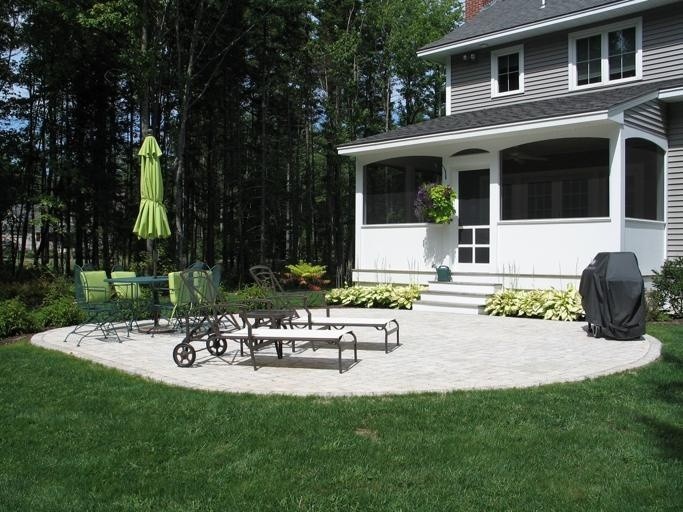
[172,269,357,374]
[64,259,242,347]
[239,264,400,354]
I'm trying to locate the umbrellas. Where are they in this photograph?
[130,128,173,278]
